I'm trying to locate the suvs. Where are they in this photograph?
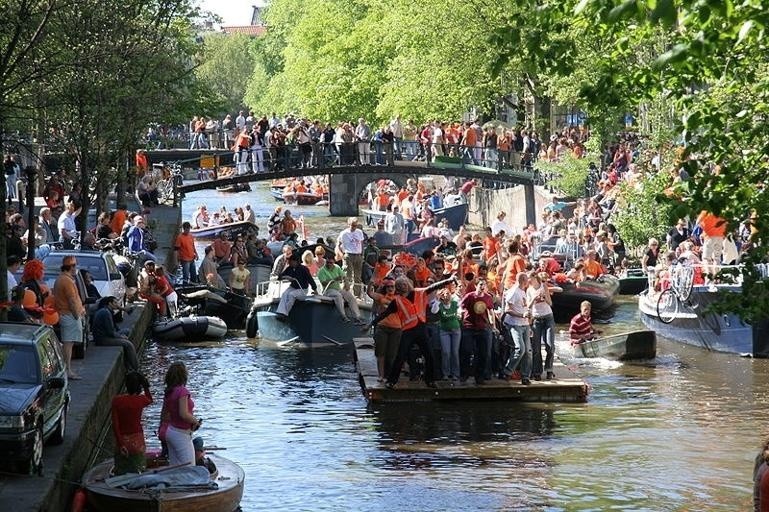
[0,322,72,478]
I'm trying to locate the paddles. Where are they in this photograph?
[104,461,191,488]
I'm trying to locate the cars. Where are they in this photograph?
[13,251,125,352]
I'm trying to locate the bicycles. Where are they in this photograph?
[656,269,697,323]
[157,176,182,205]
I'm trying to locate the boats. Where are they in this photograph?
[152,182,648,348]
[574,329,658,357]
[78,447,245,511]
[349,336,589,405]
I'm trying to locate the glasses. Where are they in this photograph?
[387,285,394,288]
[238,262,245,265]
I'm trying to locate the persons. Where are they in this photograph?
[361,112,603,390]
[751,436,769,512]
[110,370,154,475]
[192,436,210,470]
[159,359,203,468]
[581,111,768,293]
[1,108,374,382]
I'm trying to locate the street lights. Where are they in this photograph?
[22,134,42,260]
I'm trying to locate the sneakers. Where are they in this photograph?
[379,371,554,389]
[340,317,369,326]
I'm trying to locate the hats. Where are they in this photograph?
[183,222,192,228]
[386,280,395,286]
[134,216,144,223]
[63,256,76,266]
